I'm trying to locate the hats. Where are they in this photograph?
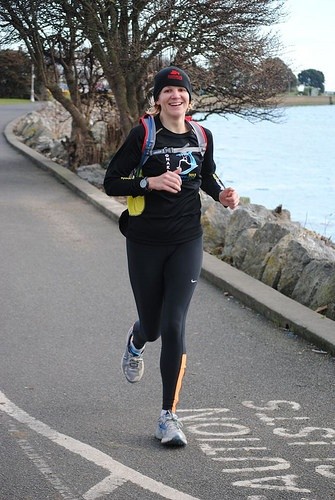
[153,66,191,104]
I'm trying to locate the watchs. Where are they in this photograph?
[139,176,153,192]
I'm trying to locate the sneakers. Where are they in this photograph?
[121,326,144,383]
[155,413,187,447]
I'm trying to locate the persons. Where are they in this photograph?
[101,66,242,450]
